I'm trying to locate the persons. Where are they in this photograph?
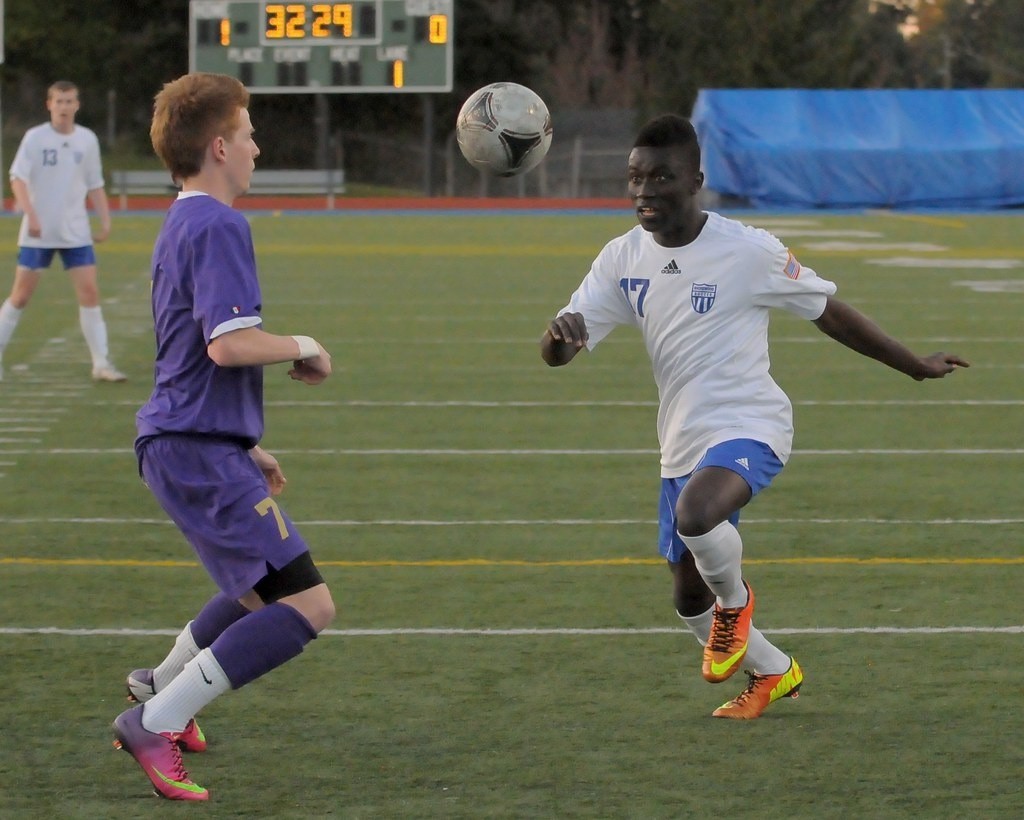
[541,120,970,717]
[105,74,335,800]
[0,81,127,382]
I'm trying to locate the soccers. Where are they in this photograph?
[455,80,555,180]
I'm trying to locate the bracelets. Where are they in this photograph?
[292,335,320,360]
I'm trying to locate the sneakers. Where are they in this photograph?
[111,703,209,800]
[702,578,754,683]
[712,656,804,719]
[91,366,125,382]
[126,669,206,752]
